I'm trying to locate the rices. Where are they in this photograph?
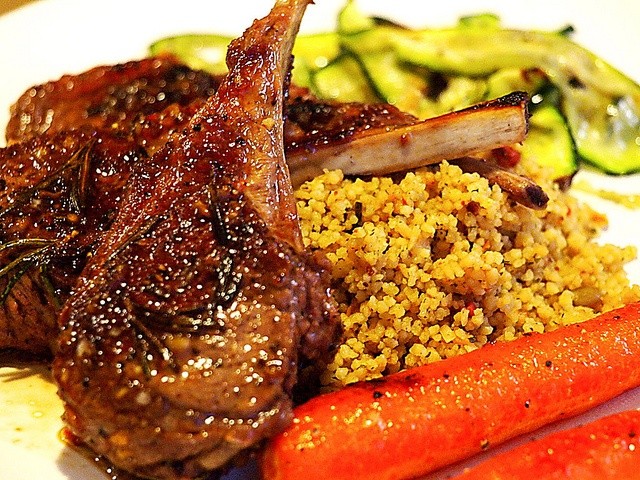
[293,159,639,391]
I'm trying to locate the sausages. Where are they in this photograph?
[264,301,639,478]
